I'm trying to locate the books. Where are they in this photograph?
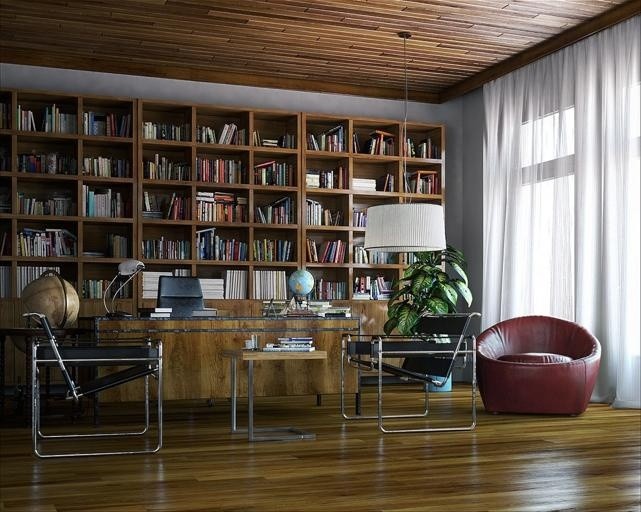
[323,307,352,313]
[280,341,312,344]
[261,300,288,317]
[325,313,352,318]
[263,347,315,352]
[137,308,173,313]
[403,134,441,299]
[140,313,171,318]
[353,130,399,299]
[141,121,192,298]
[306,125,348,299]
[277,337,314,341]
[16,103,79,298]
[281,345,311,348]
[1,103,12,299]
[83,110,134,299]
[310,305,333,307]
[196,123,249,299]
[308,301,330,305]
[253,130,298,299]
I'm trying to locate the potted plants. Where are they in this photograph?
[383,245,473,393]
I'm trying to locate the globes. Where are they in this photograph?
[20,270,81,330]
[287,269,317,318]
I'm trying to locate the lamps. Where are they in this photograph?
[104,259,143,321]
[364,31,446,253]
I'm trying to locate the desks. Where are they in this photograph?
[0,328,94,424]
[221,350,327,440]
[78,315,361,415]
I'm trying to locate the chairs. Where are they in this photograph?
[24,314,163,458]
[159,275,203,317]
[339,313,480,433]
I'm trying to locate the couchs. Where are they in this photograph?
[478,315,602,415]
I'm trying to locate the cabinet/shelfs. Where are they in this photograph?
[0,86,137,300]
[138,99,301,298]
[301,111,445,300]
